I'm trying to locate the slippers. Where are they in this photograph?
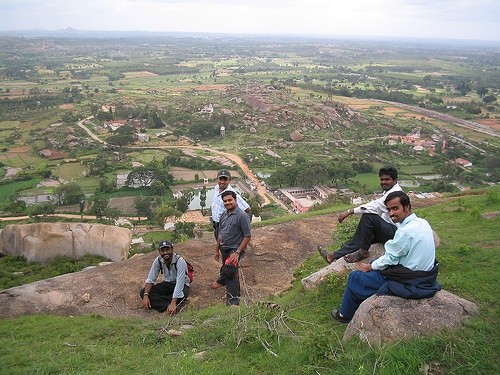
[317,245,332,263]
[344,249,368,263]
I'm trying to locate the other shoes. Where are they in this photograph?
[331,309,350,323]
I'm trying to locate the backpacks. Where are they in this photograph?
[158,254,194,284]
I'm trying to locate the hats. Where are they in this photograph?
[217,169,230,178]
[158,240,172,249]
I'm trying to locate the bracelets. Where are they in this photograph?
[235,249,241,254]
[346,209,351,215]
[143,293,148,296]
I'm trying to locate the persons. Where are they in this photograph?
[139,239,191,314]
[318,164,410,263]
[209,190,251,306]
[331,191,442,321]
[210,170,253,262]
